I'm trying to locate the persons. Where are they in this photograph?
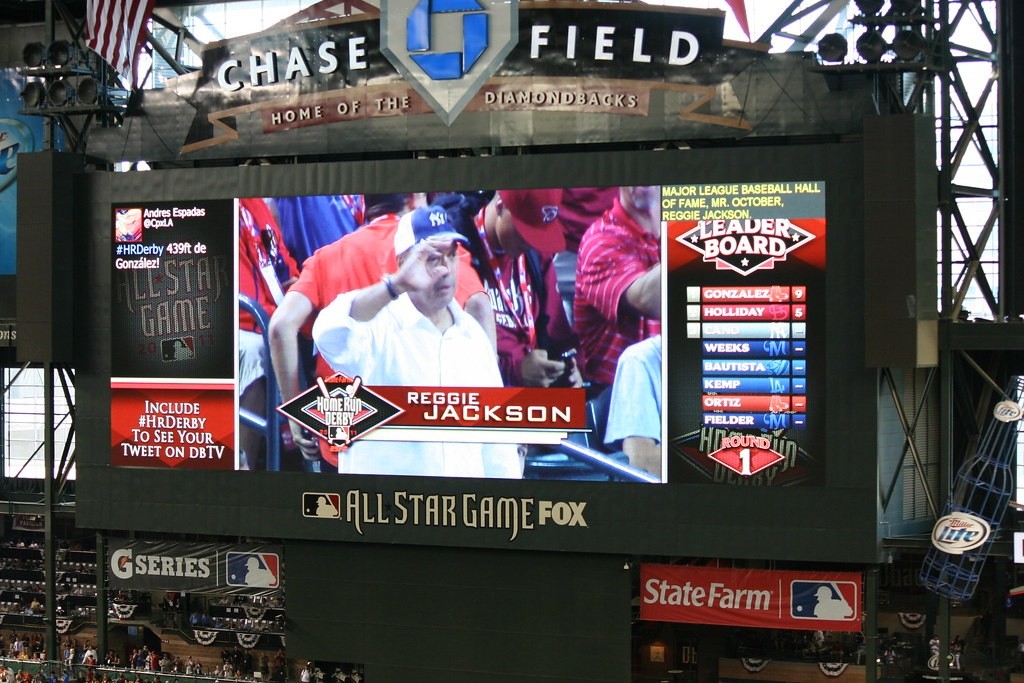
[472,189,585,388]
[574,187,660,400]
[928,635,940,667]
[311,203,527,479]
[811,630,825,649]
[269,193,498,472]
[603,334,663,476]
[884,646,896,664]
[950,635,962,669]
[239,197,315,470]
[0,538,363,683]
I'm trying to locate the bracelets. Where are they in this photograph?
[382,274,399,301]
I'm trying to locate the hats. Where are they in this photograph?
[495,187,568,254]
[392,205,470,259]
[361,193,407,223]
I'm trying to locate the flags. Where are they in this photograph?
[83,0,156,94]
